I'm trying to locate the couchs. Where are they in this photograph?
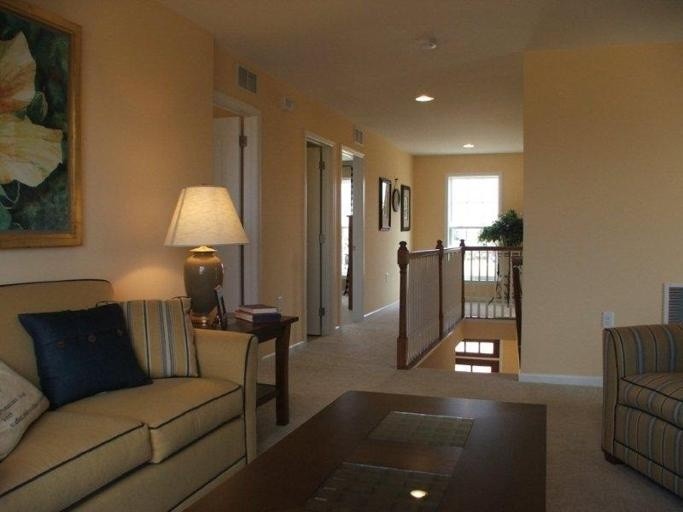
[0,278,258,511]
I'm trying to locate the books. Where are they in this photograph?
[237,303,278,315]
[233,309,281,324]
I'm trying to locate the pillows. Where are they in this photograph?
[0,296,199,462]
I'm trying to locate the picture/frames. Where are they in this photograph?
[0,0,83,251]
[378,177,410,232]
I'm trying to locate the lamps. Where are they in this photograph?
[162,185,250,328]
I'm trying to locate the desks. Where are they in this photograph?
[183,390,547,512]
[210,312,299,427]
